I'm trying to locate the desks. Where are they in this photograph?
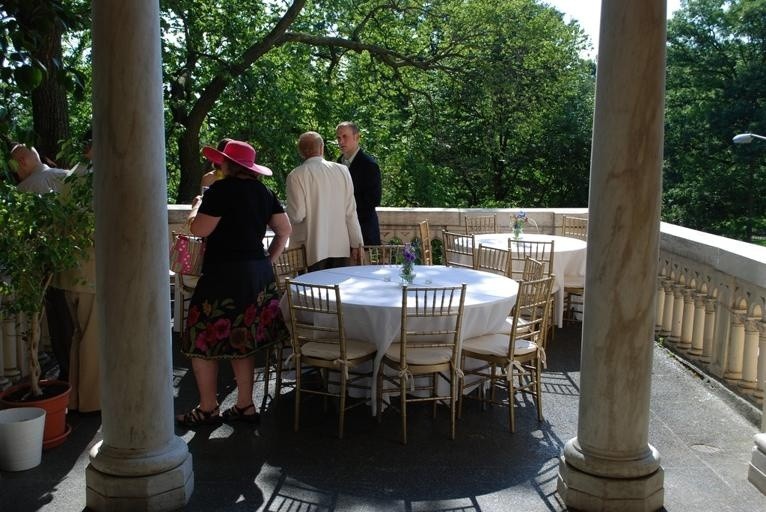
[450,232,587,329]
[173,232,292,336]
[278,264,520,417]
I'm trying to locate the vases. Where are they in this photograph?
[512,236,524,247]
[0,407,47,471]
[399,274,417,284]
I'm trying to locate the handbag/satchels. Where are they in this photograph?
[169,215,204,277]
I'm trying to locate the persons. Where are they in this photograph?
[286,130,364,272]
[336,121,382,251]
[53,127,103,422]
[200,138,232,189]
[175,140,292,426]
[8,143,70,367]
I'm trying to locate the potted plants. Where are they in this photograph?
[0,133,104,450]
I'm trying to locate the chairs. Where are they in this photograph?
[465,213,496,235]
[507,236,561,350]
[456,273,557,434]
[259,243,329,415]
[285,276,378,440]
[375,281,467,445]
[418,220,433,266]
[359,243,412,266]
[441,229,477,269]
[477,243,512,277]
[477,254,546,408]
[562,215,588,328]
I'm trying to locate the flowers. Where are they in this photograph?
[509,209,529,236]
[401,242,417,284]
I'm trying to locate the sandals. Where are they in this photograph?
[220,403,260,424]
[176,406,223,426]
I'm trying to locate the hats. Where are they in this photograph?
[202,141,273,177]
